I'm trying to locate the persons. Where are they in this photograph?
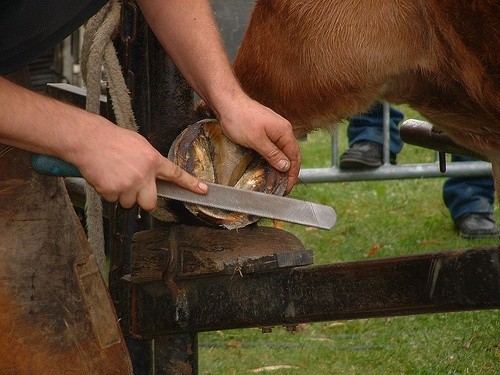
[0,0,302,375]
[340,102,499,237]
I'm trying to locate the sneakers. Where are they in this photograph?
[340,138,396,169]
[457,213,500,239]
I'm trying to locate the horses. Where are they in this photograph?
[168,0,500,231]
